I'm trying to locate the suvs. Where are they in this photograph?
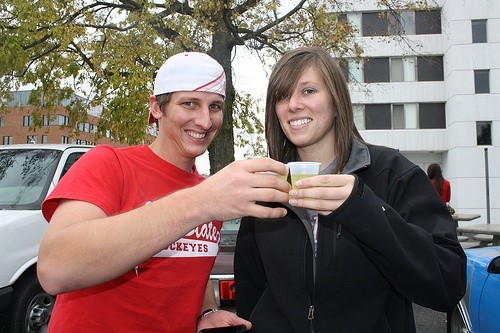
[0,143,98,333]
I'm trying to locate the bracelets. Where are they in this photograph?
[196,309,216,328]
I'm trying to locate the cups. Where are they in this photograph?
[255,164,289,182]
[289,162,321,190]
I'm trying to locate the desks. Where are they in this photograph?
[457,224,500,246]
[452,213,481,227]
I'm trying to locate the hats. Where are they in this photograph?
[147,51,226,125]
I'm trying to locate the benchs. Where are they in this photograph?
[457,234,493,249]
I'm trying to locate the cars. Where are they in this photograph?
[445,245,499,333]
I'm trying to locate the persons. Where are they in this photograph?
[234,47,467,333]
[427,163,450,206]
[37,52,291,333]
